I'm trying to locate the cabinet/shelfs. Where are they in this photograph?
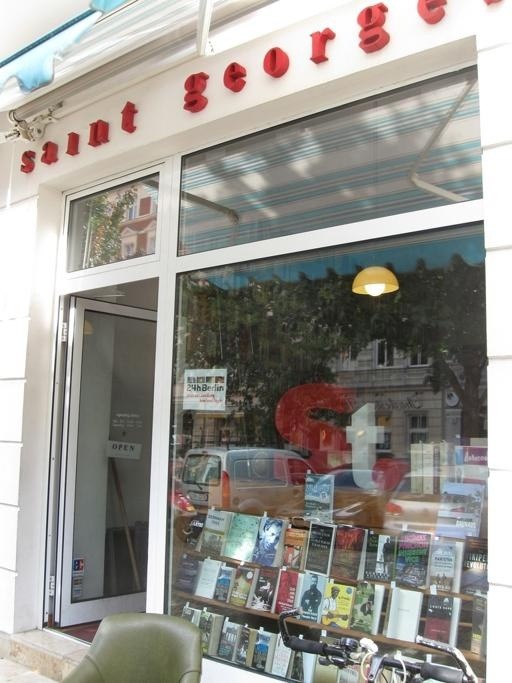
[167,506,485,666]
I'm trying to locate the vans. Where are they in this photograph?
[172,445,319,539]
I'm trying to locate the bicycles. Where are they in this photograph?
[273,607,478,682]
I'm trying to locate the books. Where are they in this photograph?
[171,437,484,683]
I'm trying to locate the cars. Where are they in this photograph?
[382,463,488,557]
[300,466,399,532]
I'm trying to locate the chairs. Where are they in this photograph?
[61,612,205,682]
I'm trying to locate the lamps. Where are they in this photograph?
[350,261,400,298]
